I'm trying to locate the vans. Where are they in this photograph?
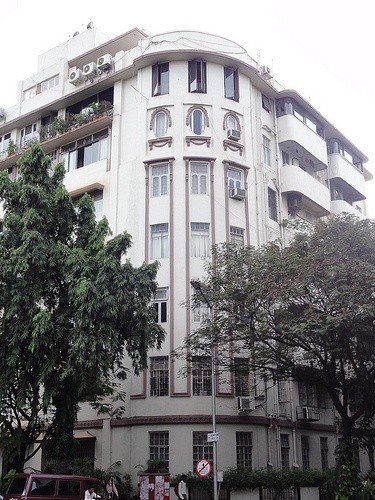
[2,472,120,500]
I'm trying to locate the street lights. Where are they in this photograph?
[190,280,216,500]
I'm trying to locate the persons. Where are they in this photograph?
[84,486,97,500]
[178,474,188,500]
